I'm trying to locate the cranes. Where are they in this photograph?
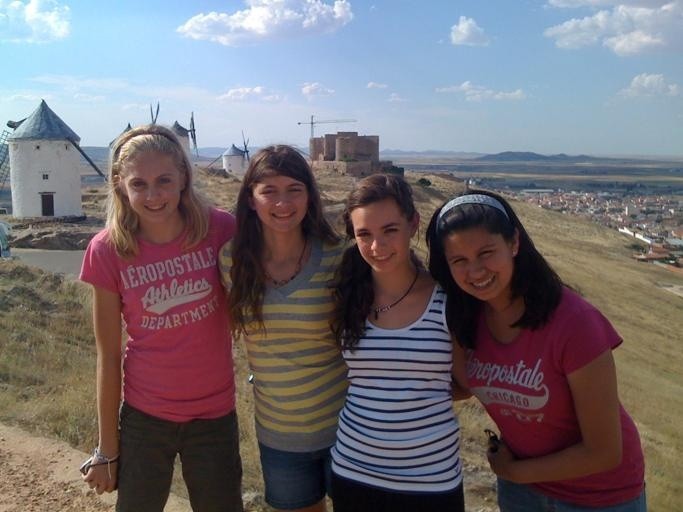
[298,115,357,137]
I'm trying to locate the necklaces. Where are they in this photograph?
[253,229,310,286]
[367,256,422,320]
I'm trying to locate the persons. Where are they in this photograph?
[80,125,249,511]
[426,191,651,512]
[326,177,467,511]
[213,141,347,512]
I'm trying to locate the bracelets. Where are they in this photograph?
[93,447,118,465]
